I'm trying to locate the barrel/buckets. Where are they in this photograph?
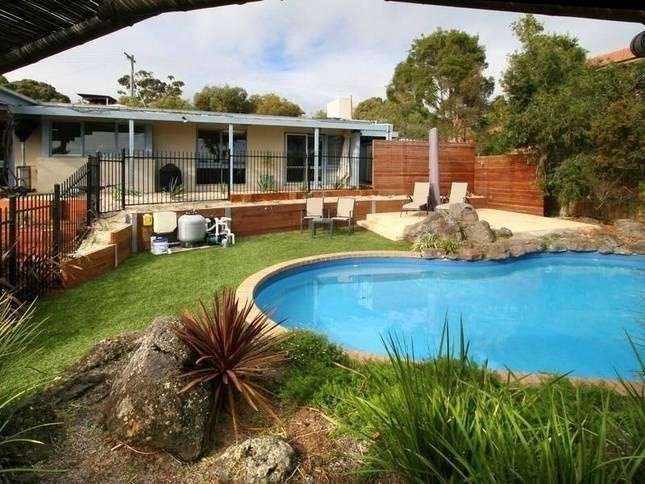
[152,236,168,255]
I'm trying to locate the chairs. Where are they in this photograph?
[400,181,468,220]
[300,196,355,239]
